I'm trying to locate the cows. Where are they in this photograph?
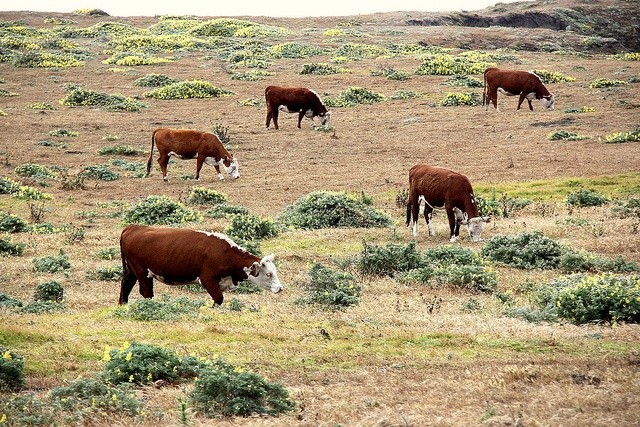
[118,224,284,310]
[483,67,555,113]
[264,85,331,130]
[405,164,491,243]
[146,127,240,182]
